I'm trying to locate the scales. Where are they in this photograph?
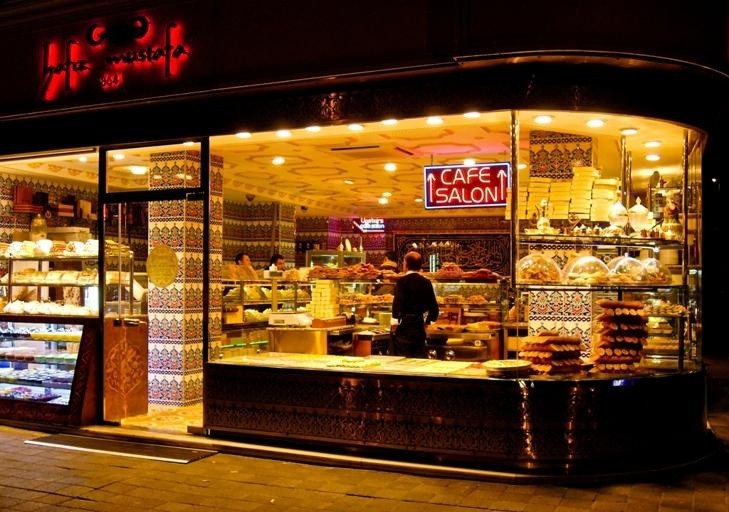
[268,312,312,326]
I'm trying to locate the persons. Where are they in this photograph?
[257,255,287,312]
[363,250,401,296]
[391,252,439,357]
[223,252,259,310]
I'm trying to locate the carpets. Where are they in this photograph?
[23,433,219,464]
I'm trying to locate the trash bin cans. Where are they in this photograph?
[351,331,376,356]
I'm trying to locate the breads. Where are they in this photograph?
[517,298,687,376]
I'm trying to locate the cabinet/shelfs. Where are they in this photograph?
[0,252,134,319]
[221,280,513,357]
[0,314,99,426]
[515,236,686,291]
[647,184,702,298]
